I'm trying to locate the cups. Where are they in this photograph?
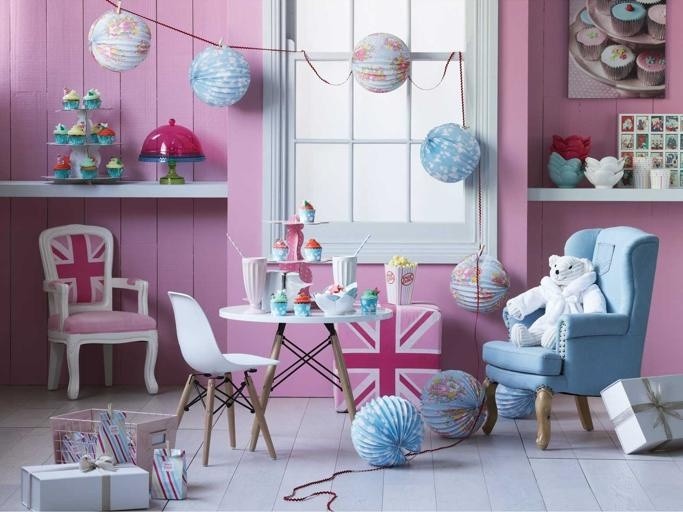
[332,256,357,287]
[242,257,267,314]
[649,168,671,190]
[632,156,653,189]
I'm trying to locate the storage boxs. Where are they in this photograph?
[600,373,682,454]
[49,409,178,490]
[20,459,150,511]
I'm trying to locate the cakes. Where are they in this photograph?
[83,88,102,110]
[52,153,124,179]
[303,239,322,261]
[300,199,316,223]
[575,0,666,86]
[62,86,80,110]
[272,238,289,261]
[293,292,312,316]
[270,290,288,316]
[53,121,116,145]
[360,287,381,312]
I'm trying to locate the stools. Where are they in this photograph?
[333,301,442,413]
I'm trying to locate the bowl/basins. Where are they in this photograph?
[310,281,358,315]
[584,157,625,190]
[547,135,592,188]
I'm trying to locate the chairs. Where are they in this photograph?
[39,224,160,400]
[164,291,277,468]
[481,225,659,451]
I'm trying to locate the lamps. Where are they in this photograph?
[139,118,207,184]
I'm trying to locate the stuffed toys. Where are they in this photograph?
[505,254,608,350]
